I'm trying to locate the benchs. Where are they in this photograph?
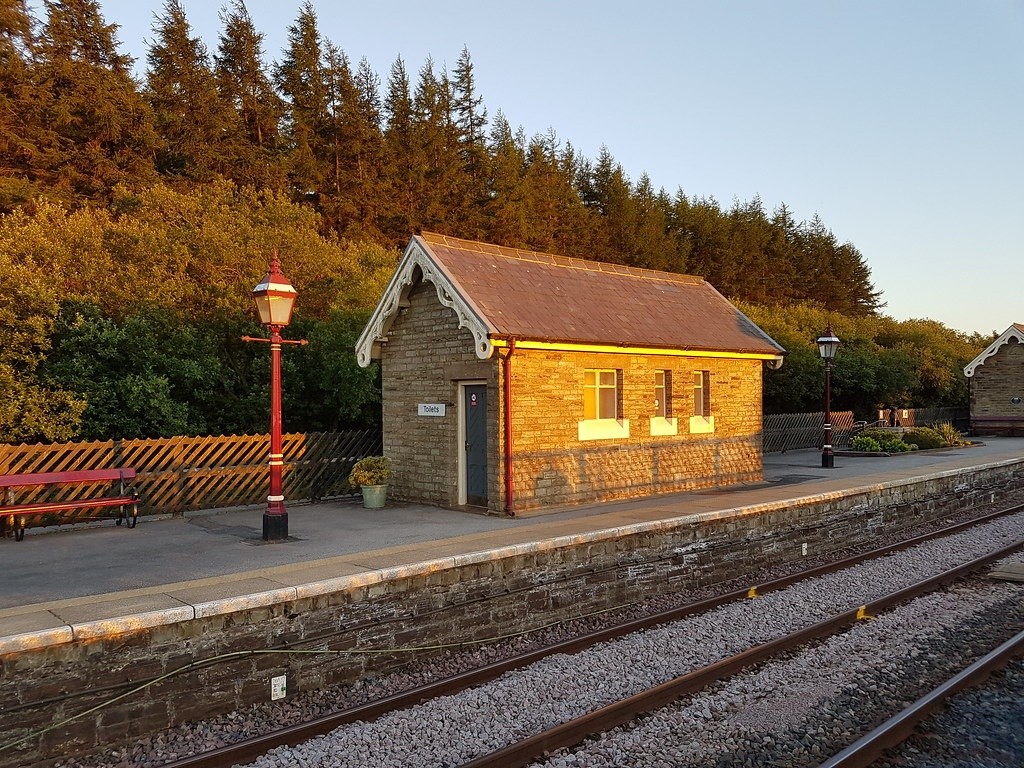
[970,416,1024,437]
[0,468,143,543]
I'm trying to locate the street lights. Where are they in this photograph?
[239,246,309,545]
[815,320,841,469]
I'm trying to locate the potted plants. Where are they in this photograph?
[349,455,394,509]
